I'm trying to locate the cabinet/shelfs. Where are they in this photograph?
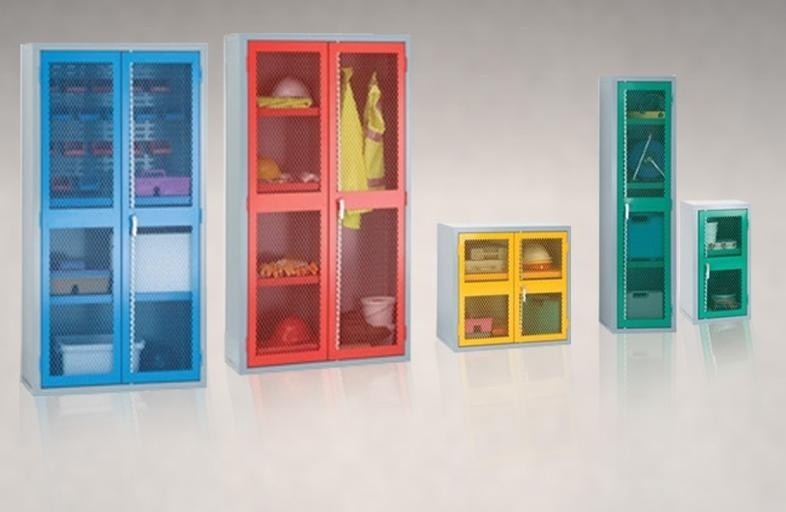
[598,73,677,335]
[681,200,750,323]
[224,32,414,375]
[437,223,573,353]
[20,41,209,398]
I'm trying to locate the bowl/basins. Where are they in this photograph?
[523,243,555,270]
[272,76,310,97]
